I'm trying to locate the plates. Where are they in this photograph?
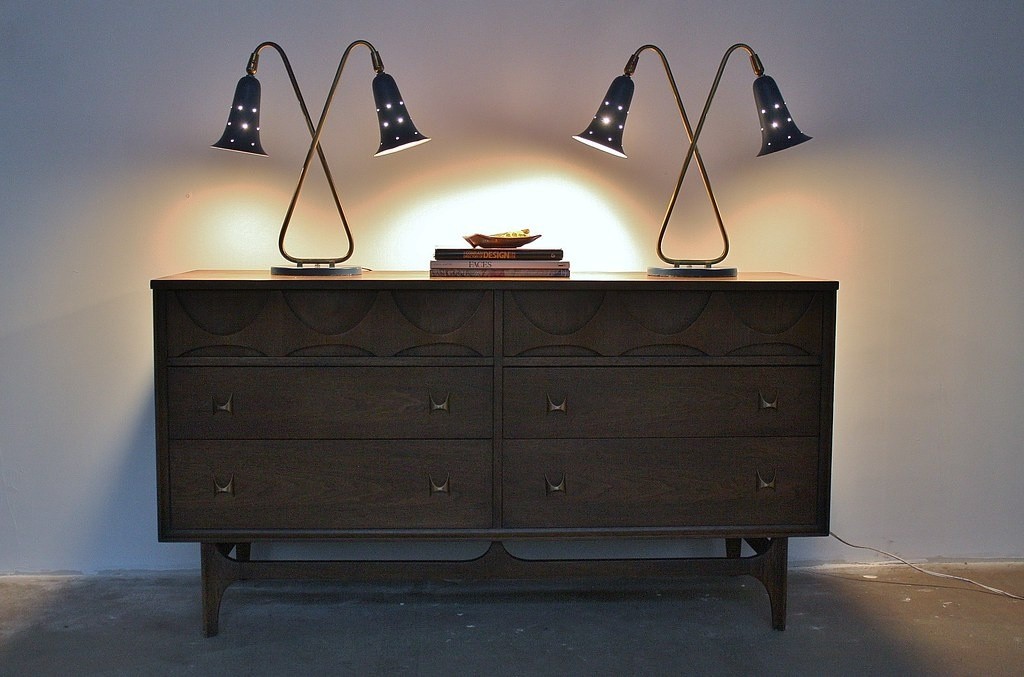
[463,234,542,248]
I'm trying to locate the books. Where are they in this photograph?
[430,248,570,278]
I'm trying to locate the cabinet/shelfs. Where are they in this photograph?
[149,269,840,640]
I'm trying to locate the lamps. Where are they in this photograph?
[571,43,813,278]
[212,41,430,276]
